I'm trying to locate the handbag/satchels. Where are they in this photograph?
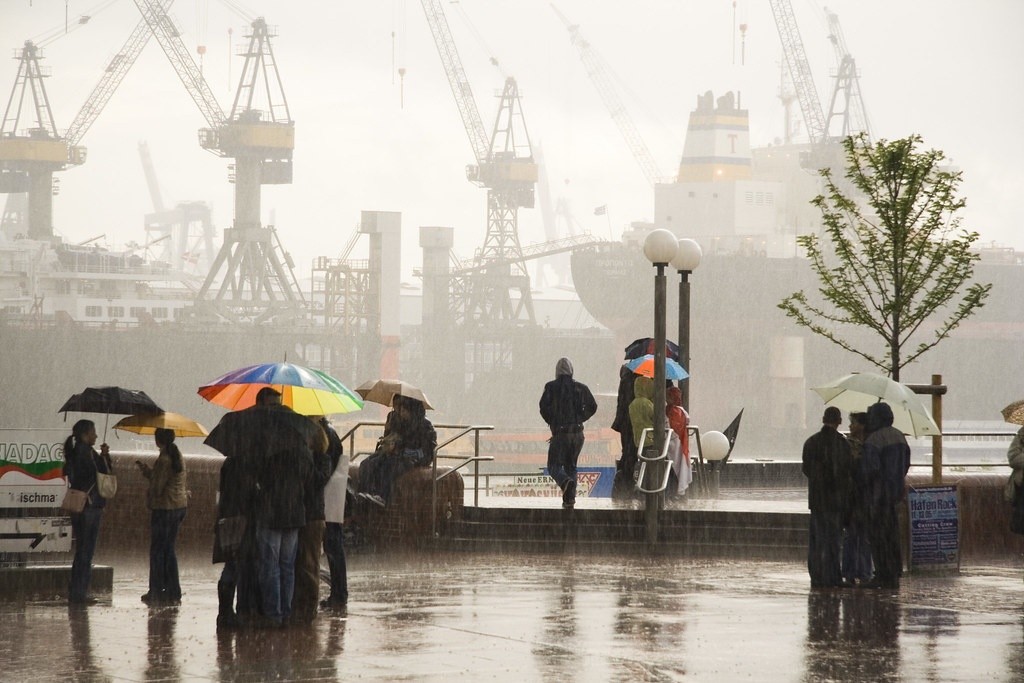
[60,488,89,513]
[1003,474,1016,506]
[211,517,256,564]
[90,448,117,499]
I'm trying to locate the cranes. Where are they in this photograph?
[134,0,299,331]
[420,0,545,415]
[135,144,215,280]
[769,0,877,173]
[0,0,176,243]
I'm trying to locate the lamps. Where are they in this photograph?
[700,430,730,500]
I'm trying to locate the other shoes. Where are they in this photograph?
[811,580,900,590]
[320,599,348,607]
[358,492,387,510]
[140,592,182,601]
[68,596,97,604]
[217,612,320,630]
[561,479,576,508]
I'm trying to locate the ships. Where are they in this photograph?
[568,0,1024,419]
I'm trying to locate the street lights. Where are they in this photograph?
[668,239,703,451]
[645,231,678,512]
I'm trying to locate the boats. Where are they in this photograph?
[905,419,1024,464]
[330,405,469,469]
[463,427,623,476]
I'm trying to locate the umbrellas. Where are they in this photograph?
[356,379,434,411]
[57,385,164,444]
[810,370,940,440]
[624,337,679,360]
[198,352,365,414]
[626,353,689,380]
[113,412,210,437]
[1002,400,1024,426]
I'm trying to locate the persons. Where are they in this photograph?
[206,389,437,631]
[802,404,910,587]
[136,428,187,600]
[611,365,691,504]
[539,358,598,508]
[1007,429,1024,535]
[64,419,113,604]
